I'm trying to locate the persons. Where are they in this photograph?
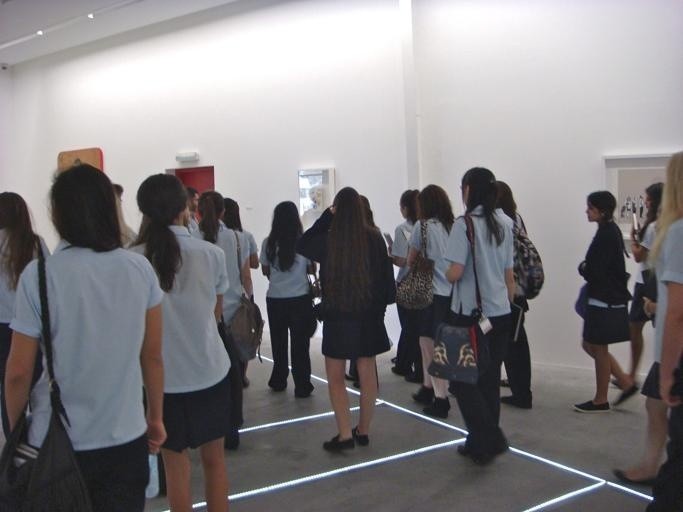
[612,151,682,511]
[572,191,642,413]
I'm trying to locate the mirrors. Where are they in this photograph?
[297,168,335,235]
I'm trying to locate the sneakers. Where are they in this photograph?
[410,385,451,420]
[573,282,597,325]
[388,356,402,378]
[499,376,533,411]
[455,428,509,465]
[344,374,362,388]
[611,467,656,488]
[292,384,316,399]
[574,400,611,416]
[322,427,370,454]
[269,379,286,393]
[608,377,641,406]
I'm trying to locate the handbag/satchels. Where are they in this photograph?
[224,294,266,366]
[395,221,435,311]
[0,392,91,512]
[426,319,503,386]
[307,260,323,322]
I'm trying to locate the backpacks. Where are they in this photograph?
[511,214,546,302]
